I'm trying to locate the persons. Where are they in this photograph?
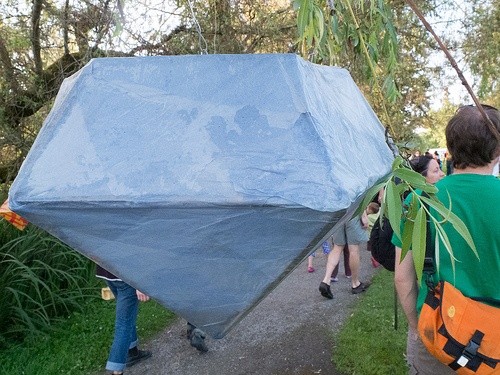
[187,322,209,352]
[95,264,152,375]
[391,104,500,375]
[308,149,448,299]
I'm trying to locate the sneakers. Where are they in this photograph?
[319,282,334,300]
[351,282,371,294]
[187,322,210,352]
[125,349,153,367]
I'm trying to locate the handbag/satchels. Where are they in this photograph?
[416,279,500,375]
[366,214,395,271]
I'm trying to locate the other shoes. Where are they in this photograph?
[307,267,315,272]
[370,256,379,267]
[345,275,352,278]
[330,277,339,282]
